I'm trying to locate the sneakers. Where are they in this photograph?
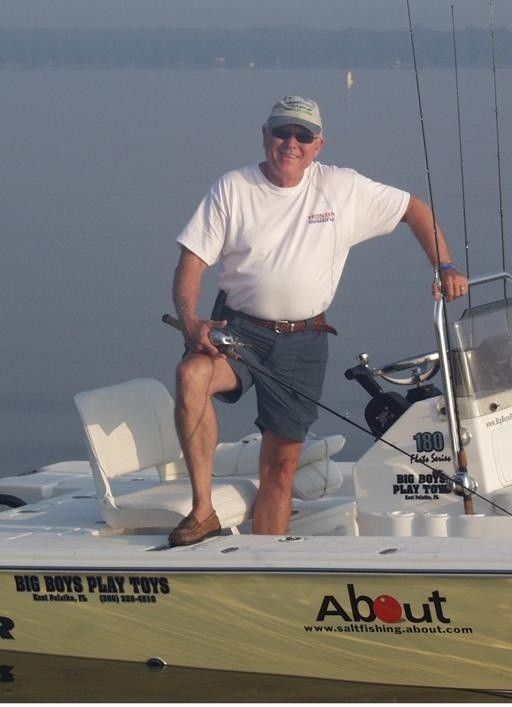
[169,510,222,546]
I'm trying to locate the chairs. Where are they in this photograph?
[70,375,262,534]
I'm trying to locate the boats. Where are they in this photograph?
[1,269,511,694]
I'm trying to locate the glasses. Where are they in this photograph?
[271,128,319,143]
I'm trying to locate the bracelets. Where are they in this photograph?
[432,261,456,274]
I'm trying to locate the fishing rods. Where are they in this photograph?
[162,314,511,516]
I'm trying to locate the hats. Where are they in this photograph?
[267,95,323,136]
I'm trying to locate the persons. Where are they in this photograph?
[161,91,471,550]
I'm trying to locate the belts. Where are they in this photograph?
[222,306,337,336]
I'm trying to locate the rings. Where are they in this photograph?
[460,286,465,290]
